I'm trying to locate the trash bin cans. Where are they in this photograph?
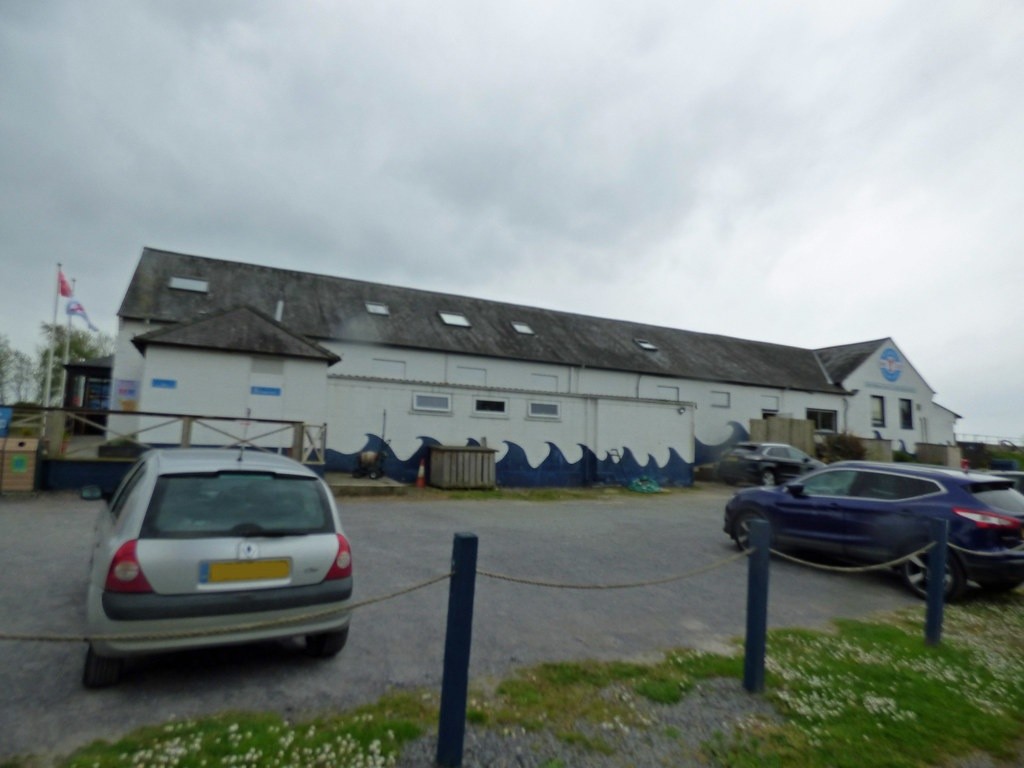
[428,445,499,490]
[0,438,42,492]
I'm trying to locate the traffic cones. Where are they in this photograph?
[416,457,425,489]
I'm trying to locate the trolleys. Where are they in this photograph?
[353,440,391,478]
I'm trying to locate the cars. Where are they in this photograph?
[85,447,353,687]
[724,461,1024,603]
[719,441,825,486]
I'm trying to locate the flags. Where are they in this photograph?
[59,272,72,297]
[67,301,90,326]
